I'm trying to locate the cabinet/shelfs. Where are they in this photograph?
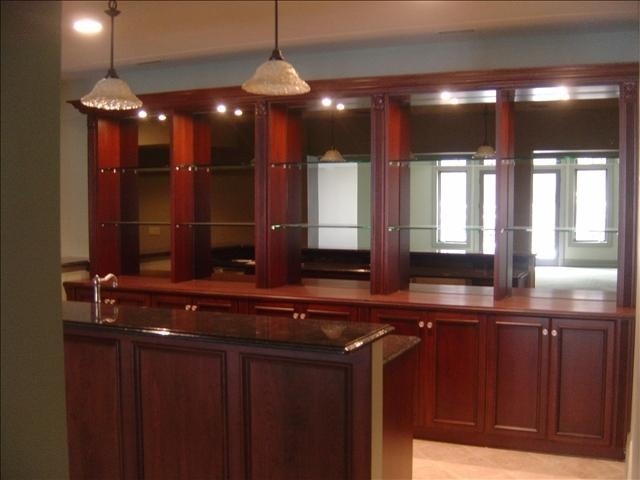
[195,116,255,278]
[301,110,369,285]
[160,293,236,313]
[250,303,361,322]
[63,329,124,479]
[129,340,356,478]
[517,107,616,309]
[398,105,495,294]
[75,293,153,306]
[490,316,616,453]
[368,310,485,437]
[122,118,171,278]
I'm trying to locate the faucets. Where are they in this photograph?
[89,273,118,302]
[91,302,120,325]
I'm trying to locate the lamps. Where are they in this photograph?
[81,0,312,111]
